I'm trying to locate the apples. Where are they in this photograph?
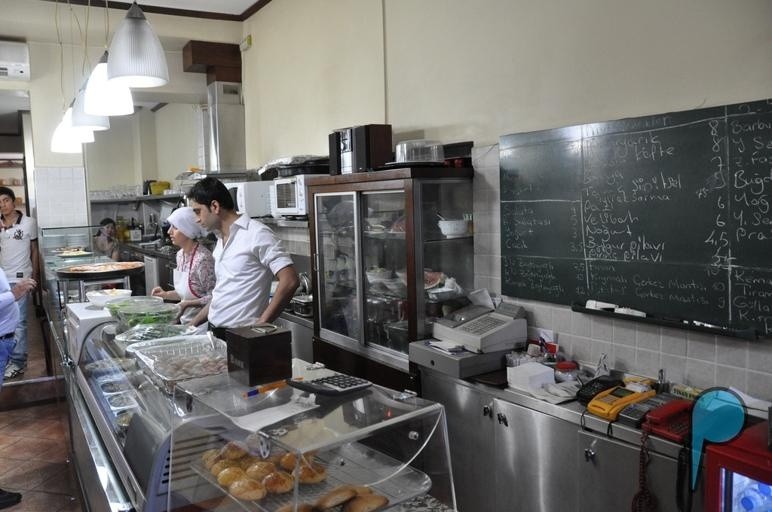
[370,266,385,273]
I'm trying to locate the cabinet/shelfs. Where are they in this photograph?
[418,371,580,512]
[311,164,475,467]
[0,157,30,216]
[67,318,233,512]
[576,426,693,512]
[166,357,458,512]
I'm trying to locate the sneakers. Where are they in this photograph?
[3,362,24,379]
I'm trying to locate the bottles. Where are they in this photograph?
[735,480,772,512]
[555,361,578,383]
[115,216,127,243]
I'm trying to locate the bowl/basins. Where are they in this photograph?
[106,295,181,323]
[365,266,461,301]
[84,288,133,308]
[437,218,470,239]
[150,182,171,195]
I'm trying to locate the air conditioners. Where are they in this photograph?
[0,39,31,82]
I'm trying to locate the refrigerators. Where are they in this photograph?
[704,421,772,512]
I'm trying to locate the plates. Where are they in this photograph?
[55,261,145,280]
[49,248,96,257]
[84,357,151,429]
[363,224,407,239]
[113,323,198,343]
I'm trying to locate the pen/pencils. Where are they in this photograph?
[243,382,286,399]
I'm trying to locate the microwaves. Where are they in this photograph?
[268,173,331,218]
[219,180,273,219]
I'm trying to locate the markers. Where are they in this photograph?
[683,319,722,329]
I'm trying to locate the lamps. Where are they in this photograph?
[50,0,170,155]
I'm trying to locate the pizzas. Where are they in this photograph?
[70,262,141,272]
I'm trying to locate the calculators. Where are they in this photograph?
[287,374,373,396]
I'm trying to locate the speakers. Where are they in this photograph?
[329,131,341,175]
[355,123,392,173]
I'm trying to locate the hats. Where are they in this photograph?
[167,205,203,239]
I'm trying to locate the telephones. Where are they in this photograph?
[587,385,649,421]
[640,399,693,444]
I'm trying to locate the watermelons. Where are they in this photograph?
[398,272,446,290]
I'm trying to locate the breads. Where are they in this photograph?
[147,352,227,379]
[201,418,389,511]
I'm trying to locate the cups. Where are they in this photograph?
[14,197,22,208]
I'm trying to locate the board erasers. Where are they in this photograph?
[585,299,647,318]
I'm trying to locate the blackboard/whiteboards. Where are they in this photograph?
[499,99,772,339]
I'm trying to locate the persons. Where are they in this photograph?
[151,206,215,325]
[0,269,37,509]
[0,186,39,380]
[92,217,121,288]
[184,175,300,341]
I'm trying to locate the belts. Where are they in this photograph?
[1,332,14,339]
